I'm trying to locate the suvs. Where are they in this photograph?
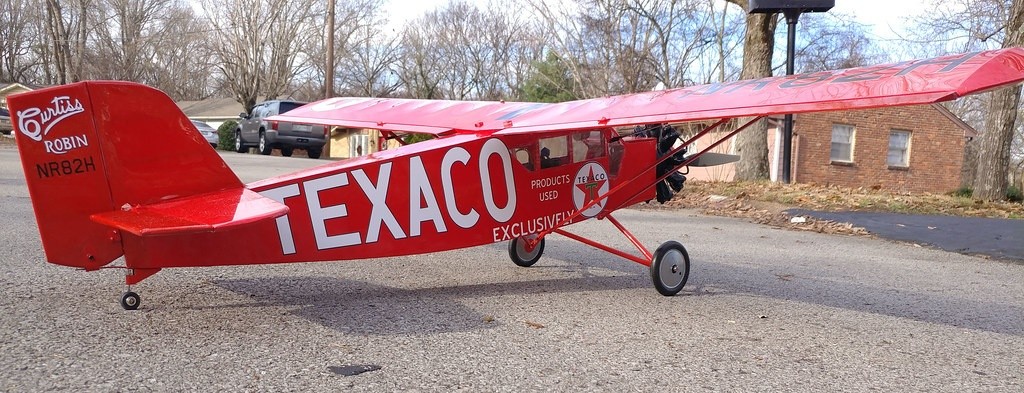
[233,100,326,157]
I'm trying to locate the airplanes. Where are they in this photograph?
[3,41,1024,312]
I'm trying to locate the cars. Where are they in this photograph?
[191,120,219,148]
[0,107,15,134]
